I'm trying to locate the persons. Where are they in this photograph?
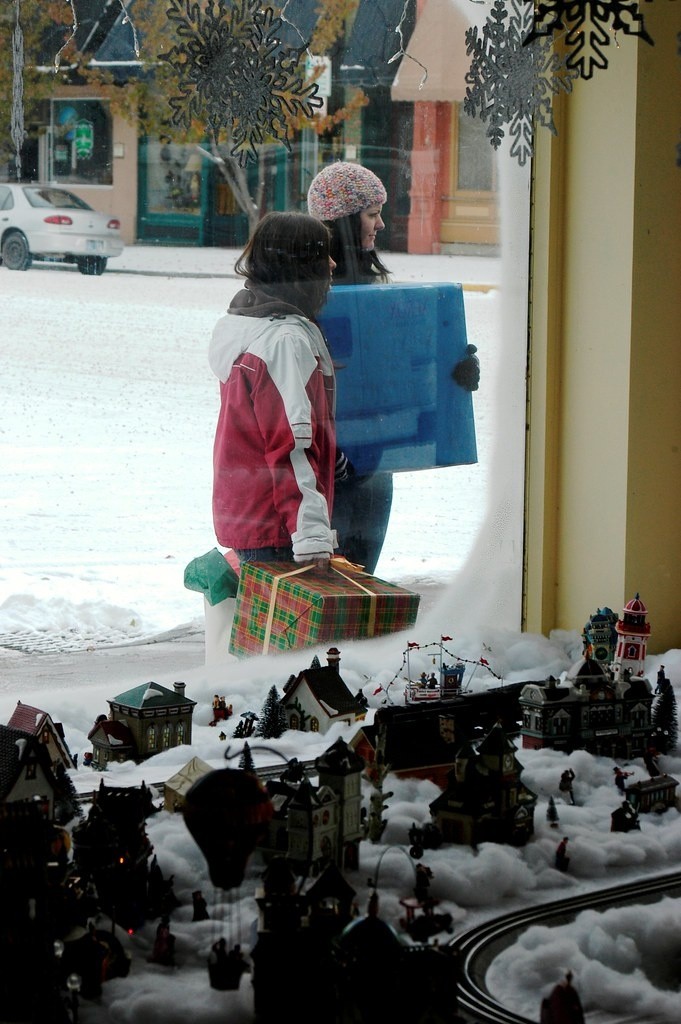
[655,665,665,694]
[212,695,226,708]
[549,972,585,1024]
[192,891,210,920]
[611,801,639,834]
[308,160,480,577]
[212,939,244,962]
[208,212,338,578]
[419,672,438,689]
[412,864,431,902]
[355,689,370,708]
[643,747,661,778]
[554,837,568,869]
[560,768,575,806]
[614,767,627,791]
[157,914,176,966]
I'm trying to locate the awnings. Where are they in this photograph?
[390,0,535,101]
[91,0,417,86]
[30,0,123,85]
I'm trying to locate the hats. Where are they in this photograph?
[307,158,387,222]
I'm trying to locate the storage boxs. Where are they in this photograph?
[314,281,478,477]
[228,554,420,658]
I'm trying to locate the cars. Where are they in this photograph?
[0,183,123,278]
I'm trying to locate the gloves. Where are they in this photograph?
[454,344,481,391]
[335,448,365,487]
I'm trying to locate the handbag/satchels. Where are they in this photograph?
[203,592,247,666]
